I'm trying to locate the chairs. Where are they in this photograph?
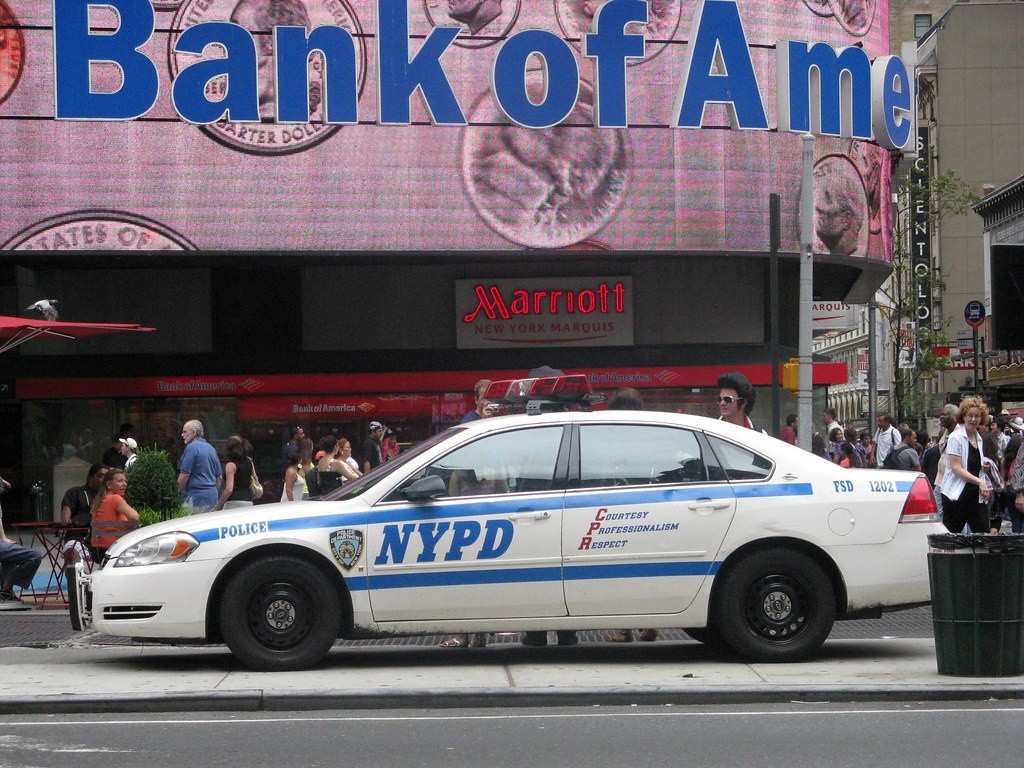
[90,521,140,569]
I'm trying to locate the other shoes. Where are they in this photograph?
[522,634,546,647]
[641,628,658,642]
[0,538,16,544]
[557,636,579,645]
[612,631,633,642]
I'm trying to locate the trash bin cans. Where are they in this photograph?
[925,533,1024,677]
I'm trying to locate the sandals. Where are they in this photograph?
[471,634,485,648]
[439,637,468,647]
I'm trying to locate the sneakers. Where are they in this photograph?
[0,585,24,604]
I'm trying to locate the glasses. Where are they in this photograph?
[963,413,981,419]
[717,394,743,404]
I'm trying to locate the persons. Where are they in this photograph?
[0,372,1024,647]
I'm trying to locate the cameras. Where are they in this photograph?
[488,404,499,413]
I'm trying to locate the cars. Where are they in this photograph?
[65,373,978,673]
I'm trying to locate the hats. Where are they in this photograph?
[315,450,326,460]
[996,409,1017,416]
[119,438,137,450]
[1009,416,1024,430]
[384,429,396,437]
[368,422,382,431]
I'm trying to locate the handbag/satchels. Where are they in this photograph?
[248,457,263,500]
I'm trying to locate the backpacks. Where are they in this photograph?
[883,444,908,470]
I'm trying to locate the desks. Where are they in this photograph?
[10,522,96,610]
